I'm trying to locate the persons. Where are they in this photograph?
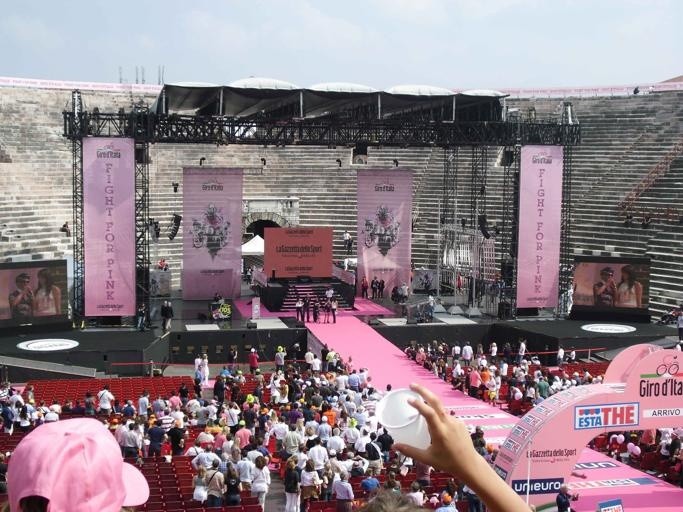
[9,273,35,318]
[62,222,71,237]
[344,230,351,250]
[215,293,224,305]
[159,258,169,271]
[8,418,150,512]
[242,266,253,285]
[593,267,618,307]
[657,304,683,352]
[34,269,61,316]
[557,485,576,512]
[471,426,500,467]
[625,213,635,227]
[642,215,651,229]
[634,86,639,94]
[1,451,13,480]
[0,381,189,458]
[161,300,167,329]
[649,86,654,94]
[165,302,173,330]
[348,236,353,254]
[590,428,683,487]
[394,383,532,512]
[361,275,408,303]
[186,347,485,512]
[295,287,338,324]
[615,265,642,307]
[429,292,435,319]
[137,303,145,328]
[405,344,604,415]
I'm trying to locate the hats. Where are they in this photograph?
[250,348,255,353]
[239,419,246,426]
[350,418,357,428]
[444,494,452,503]
[277,346,283,352]
[5,417,150,511]
[321,416,328,422]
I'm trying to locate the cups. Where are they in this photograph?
[374,388,431,453]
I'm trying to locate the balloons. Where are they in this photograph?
[617,434,624,444]
[633,446,641,455]
[627,443,634,452]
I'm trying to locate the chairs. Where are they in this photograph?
[429,346,681,512]
[0,367,440,511]
